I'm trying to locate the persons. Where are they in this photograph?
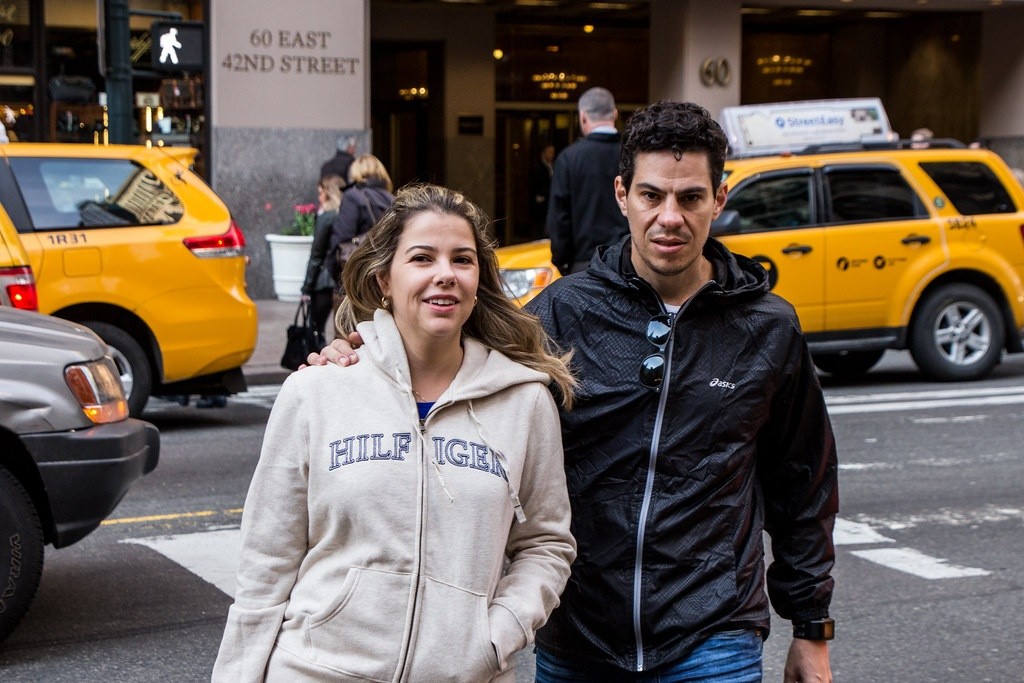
[911,129,934,150]
[209,184,578,683]
[295,98,838,683]
[301,135,395,353]
[544,87,625,277]
[528,144,555,240]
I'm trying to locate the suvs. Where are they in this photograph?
[491,98,1024,382]
[0,141,258,421]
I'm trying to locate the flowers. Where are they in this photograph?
[277,202,316,234]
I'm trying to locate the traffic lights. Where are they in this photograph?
[149,6,218,83]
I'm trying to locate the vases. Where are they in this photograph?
[263,232,315,303]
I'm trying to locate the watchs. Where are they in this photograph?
[793,618,834,640]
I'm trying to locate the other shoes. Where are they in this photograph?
[163,394,189,406]
[195,395,227,408]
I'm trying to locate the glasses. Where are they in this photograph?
[638,312,677,394]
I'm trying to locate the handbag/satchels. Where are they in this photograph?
[278,300,325,372]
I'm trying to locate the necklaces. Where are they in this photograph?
[413,389,425,402]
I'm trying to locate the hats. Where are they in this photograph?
[318,149,355,186]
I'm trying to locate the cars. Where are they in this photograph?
[0,303,161,644]
